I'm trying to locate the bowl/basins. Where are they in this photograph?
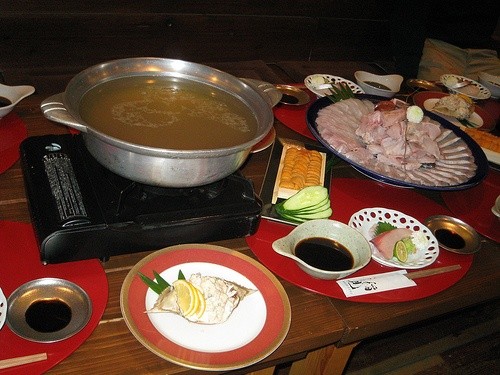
[477,71,500,99]
[354,70,404,97]
[271,218,373,281]
[0,82,36,119]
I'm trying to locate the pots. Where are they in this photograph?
[41,58,284,190]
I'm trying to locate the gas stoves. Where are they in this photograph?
[18,133,263,266]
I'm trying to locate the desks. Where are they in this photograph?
[196,59,500,375]
[0,59,345,375]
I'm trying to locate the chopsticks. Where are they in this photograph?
[404,263,462,279]
[0,353,48,370]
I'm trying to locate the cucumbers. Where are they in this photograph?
[275,185,333,223]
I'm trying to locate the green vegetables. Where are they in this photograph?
[323,82,356,101]
[138,270,186,293]
[374,221,398,235]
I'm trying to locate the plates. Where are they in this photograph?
[119,243,292,373]
[303,73,366,98]
[440,73,492,100]
[305,93,490,193]
[406,78,444,92]
[422,214,484,257]
[274,83,311,107]
[412,90,497,132]
[258,135,334,227]
[347,206,440,272]
[5,276,93,345]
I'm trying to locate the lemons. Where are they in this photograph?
[394,241,408,262]
[172,280,205,320]
